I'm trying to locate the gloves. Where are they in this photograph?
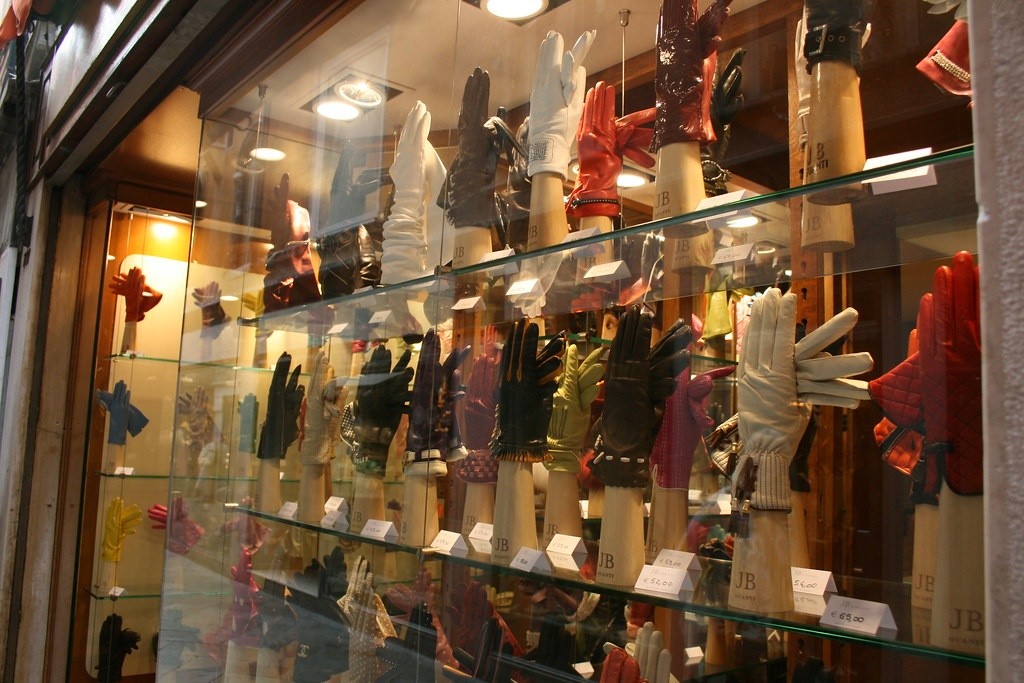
[868,252,983,508]
[381,100,432,287]
[96,614,141,682]
[351,344,415,478]
[298,350,350,466]
[190,282,231,341]
[178,386,223,483]
[403,327,472,475]
[565,81,658,219]
[647,0,734,153]
[101,497,143,563]
[794,7,870,149]
[240,279,280,369]
[108,267,163,322]
[457,351,500,483]
[438,66,530,230]
[802,0,871,69]
[234,393,259,453]
[262,171,321,311]
[524,29,597,179]
[701,287,874,510]
[582,301,694,486]
[700,260,756,342]
[315,141,392,296]
[97,379,150,444]
[702,46,747,195]
[255,351,307,460]
[543,342,605,472]
[649,317,736,488]
[145,497,895,682]
[489,317,567,463]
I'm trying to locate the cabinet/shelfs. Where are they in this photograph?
[51,146,987,683]
